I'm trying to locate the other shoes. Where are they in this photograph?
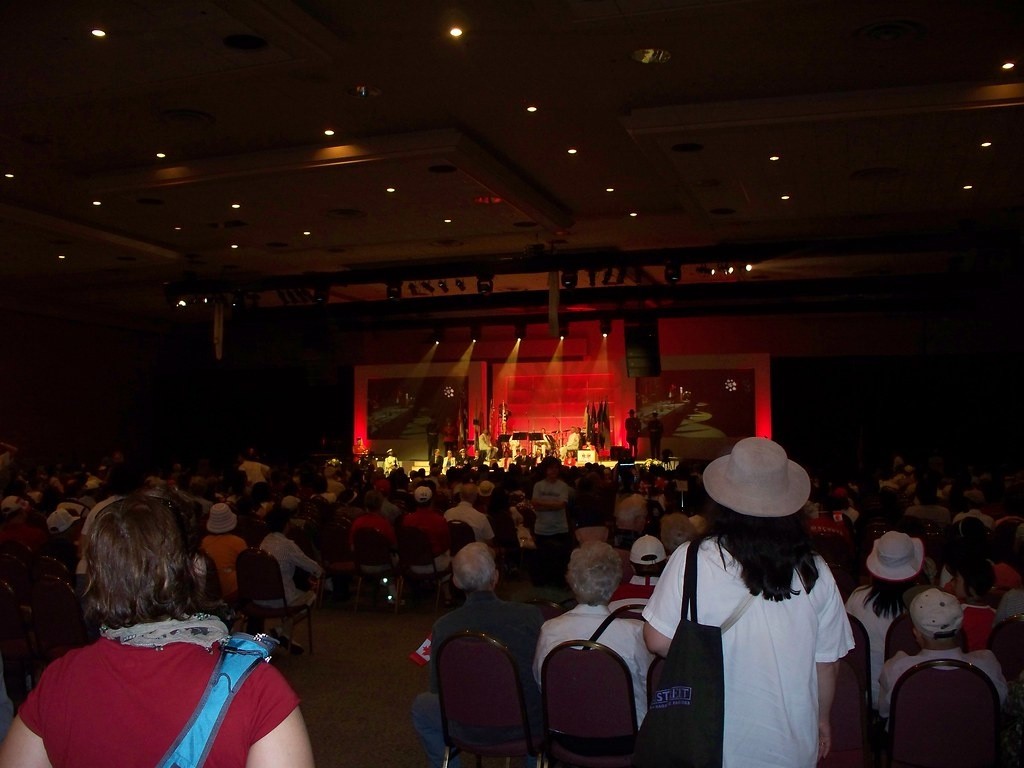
[278,635,304,656]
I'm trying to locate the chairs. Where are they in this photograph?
[0,487,1024,768]
[567,439,586,457]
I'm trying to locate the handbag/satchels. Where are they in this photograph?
[633,536,724,768]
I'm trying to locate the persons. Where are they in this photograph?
[410,542,545,768]
[642,438,854,768]
[532,541,656,757]
[0,409,1024,710]
[879,584,1008,768]
[1003,678,1024,768]
[0,491,317,768]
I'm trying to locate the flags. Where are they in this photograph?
[409,633,432,667]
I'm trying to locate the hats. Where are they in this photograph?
[83,481,100,489]
[81,476,103,484]
[1,496,29,517]
[630,534,667,565]
[703,437,811,518]
[866,531,925,582]
[281,495,301,511]
[652,413,658,417]
[207,503,237,534]
[47,509,80,534]
[415,486,432,503]
[478,481,495,496]
[903,585,963,639]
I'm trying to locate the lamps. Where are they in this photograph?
[171,261,681,346]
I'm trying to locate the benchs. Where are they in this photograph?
[411,460,457,477]
[377,460,403,478]
